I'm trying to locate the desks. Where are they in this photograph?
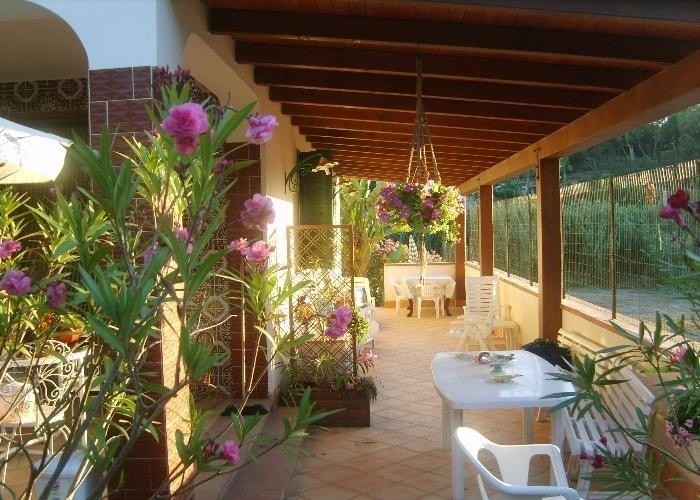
[0,348,91,452]
[430,350,577,463]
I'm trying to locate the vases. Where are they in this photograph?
[286,390,370,427]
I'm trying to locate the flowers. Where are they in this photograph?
[327,349,383,406]
[370,178,466,247]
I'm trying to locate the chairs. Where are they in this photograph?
[0,340,75,488]
[389,275,410,317]
[452,427,580,500]
[415,283,446,319]
[456,274,520,350]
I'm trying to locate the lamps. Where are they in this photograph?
[285,153,339,194]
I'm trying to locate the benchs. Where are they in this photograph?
[536,327,656,500]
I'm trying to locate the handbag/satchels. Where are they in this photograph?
[522,338,571,372]
